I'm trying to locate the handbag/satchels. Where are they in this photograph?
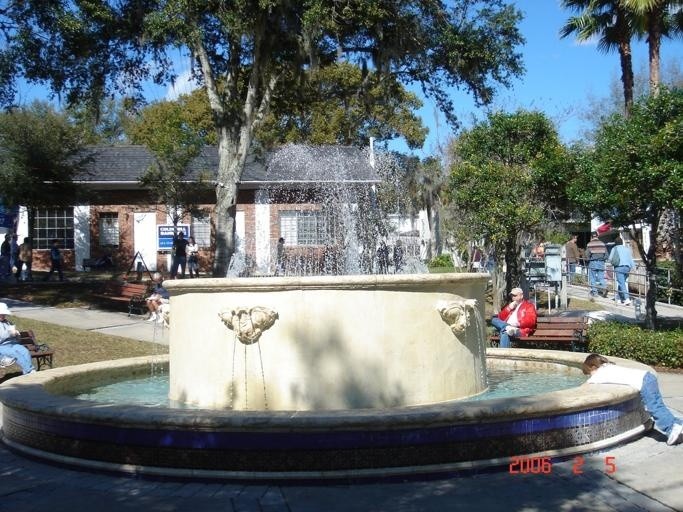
[611,246,621,267]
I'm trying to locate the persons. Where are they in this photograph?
[0,303,36,375]
[145,272,169,324]
[491,288,537,348]
[471,246,480,262]
[158,275,182,323]
[185,236,200,277]
[172,232,188,279]
[585,232,608,297]
[377,241,388,274]
[0,234,33,281]
[274,238,290,276]
[608,237,636,305]
[393,240,403,273]
[582,354,683,445]
[42,239,64,282]
[565,236,580,285]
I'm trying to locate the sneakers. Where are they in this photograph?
[667,424,683,446]
[507,327,521,337]
[146,312,164,323]
[590,288,632,306]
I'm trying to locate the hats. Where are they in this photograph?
[508,288,523,296]
[0,302,11,315]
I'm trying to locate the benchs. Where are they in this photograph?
[20,330,54,370]
[490,316,588,352]
[90,282,149,317]
